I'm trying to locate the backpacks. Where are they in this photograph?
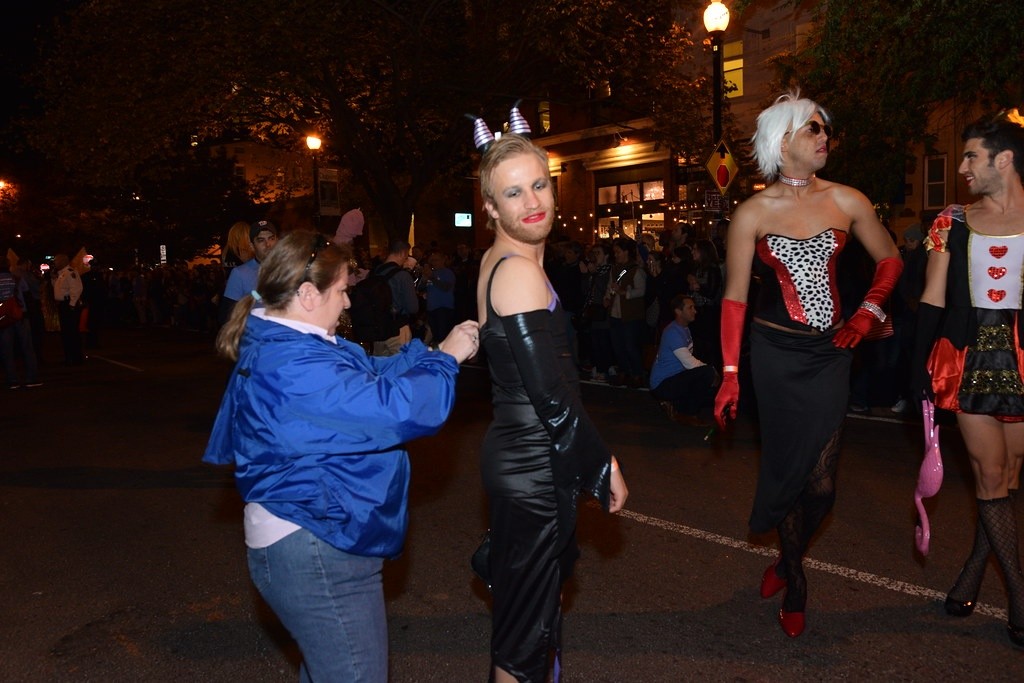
[351,262,404,341]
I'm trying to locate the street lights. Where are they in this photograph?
[307,134,323,234]
[704,0,730,149]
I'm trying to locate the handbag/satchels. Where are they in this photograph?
[862,294,894,340]
[0,296,21,329]
[583,304,607,321]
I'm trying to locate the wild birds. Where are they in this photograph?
[914,389,944,557]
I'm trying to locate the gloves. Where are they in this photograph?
[912,300,943,415]
[713,298,749,431]
[831,259,902,349]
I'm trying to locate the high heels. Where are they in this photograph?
[1006,605,1024,650]
[779,588,805,637]
[760,552,783,597]
[946,558,978,616]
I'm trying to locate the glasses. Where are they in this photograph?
[789,121,835,139]
[295,233,334,291]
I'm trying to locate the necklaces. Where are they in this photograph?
[778,172,815,187]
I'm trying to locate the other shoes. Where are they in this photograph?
[613,371,628,389]
[630,374,643,388]
[666,404,710,428]
[25,379,44,387]
[8,382,20,389]
[891,399,910,413]
[590,376,607,383]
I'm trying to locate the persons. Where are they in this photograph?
[650,294,720,427]
[87,223,255,346]
[543,220,730,388]
[200,228,480,683]
[850,213,934,414]
[908,113,1024,650]
[221,218,277,312]
[50,254,84,366]
[344,240,480,346]
[712,89,903,635]
[472,108,629,683]
[0,256,45,389]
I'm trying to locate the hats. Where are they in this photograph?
[249,220,277,243]
